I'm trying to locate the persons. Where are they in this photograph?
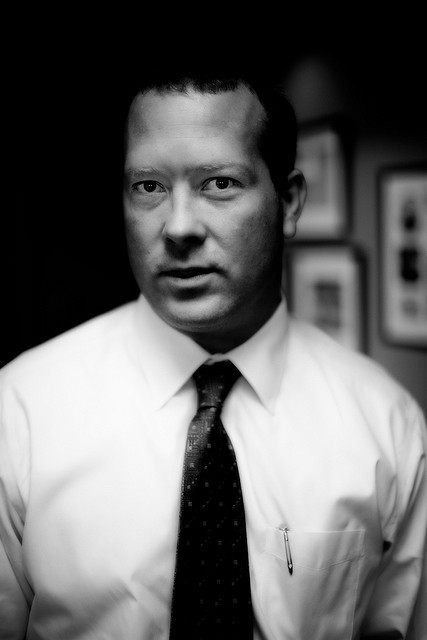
[0,55,426,640]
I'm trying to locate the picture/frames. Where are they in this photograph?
[376,164,427,348]
[280,117,352,243]
[281,242,368,356]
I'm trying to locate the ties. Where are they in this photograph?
[169,360,253,639]
